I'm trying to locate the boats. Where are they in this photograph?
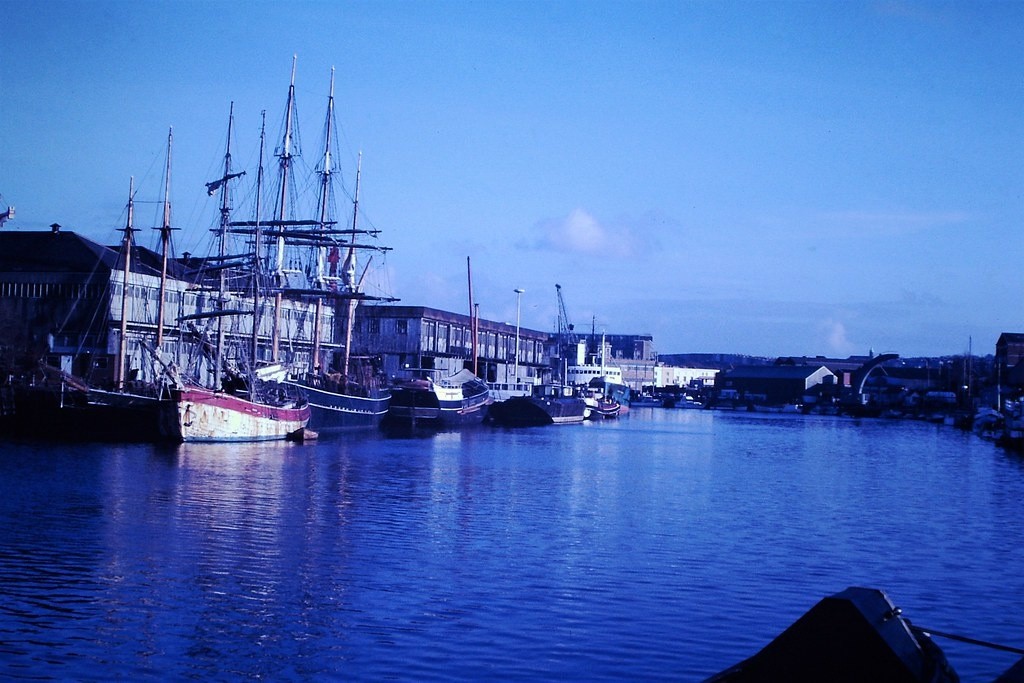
[553,330,632,409]
[388,365,489,427]
[582,393,621,419]
[489,384,587,426]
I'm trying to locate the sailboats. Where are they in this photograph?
[0,55,393,445]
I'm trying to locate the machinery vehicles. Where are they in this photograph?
[554,283,592,365]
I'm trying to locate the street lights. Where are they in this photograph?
[512,288,525,390]
